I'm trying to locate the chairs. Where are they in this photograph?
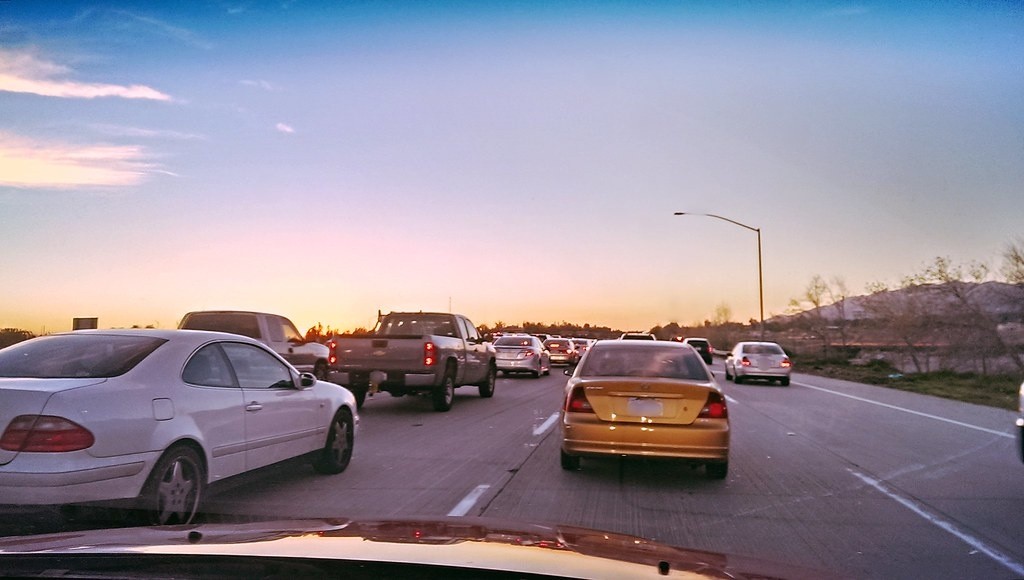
[184,354,212,384]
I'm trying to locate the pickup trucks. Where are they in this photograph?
[171,313,333,385]
[328,311,500,412]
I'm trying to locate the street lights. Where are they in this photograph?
[674,209,766,342]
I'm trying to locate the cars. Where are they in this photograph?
[689,336,712,361]
[558,340,730,478]
[0,325,358,520]
[491,335,553,378]
[534,330,610,366]
[725,343,793,387]
